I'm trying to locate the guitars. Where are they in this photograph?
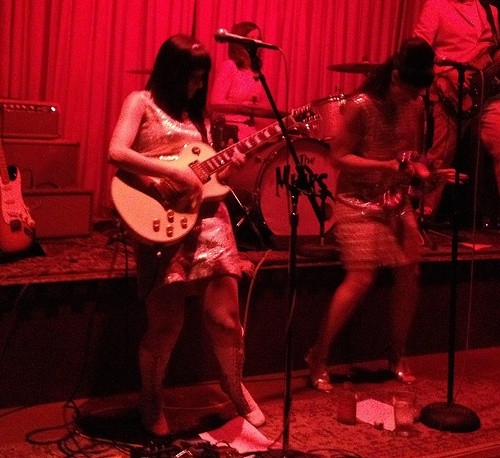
[431,41,499,119]
[0,141,41,257]
[106,103,318,252]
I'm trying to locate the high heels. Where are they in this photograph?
[389,354,415,383]
[303,349,336,393]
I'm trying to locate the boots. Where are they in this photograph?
[140,348,170,438]
[212,328,265,426]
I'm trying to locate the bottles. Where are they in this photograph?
[336,382,356,424]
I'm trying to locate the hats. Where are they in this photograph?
[388,37,435,87]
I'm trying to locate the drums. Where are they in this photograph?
[309,93,352,148]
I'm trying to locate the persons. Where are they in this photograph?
[305,37,454,394]
[212,21,263,124]
[413,0,500,184]
[108,34,266,436]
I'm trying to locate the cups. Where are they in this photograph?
[393,392,415,435]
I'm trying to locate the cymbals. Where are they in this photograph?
[219,135,339,241]
[202,102,287,120]
[325,61,387,81]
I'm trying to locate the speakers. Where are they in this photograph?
[0,99,94,237]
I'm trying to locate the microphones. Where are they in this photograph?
[433,55,482,72]
[214,28,280,50]
